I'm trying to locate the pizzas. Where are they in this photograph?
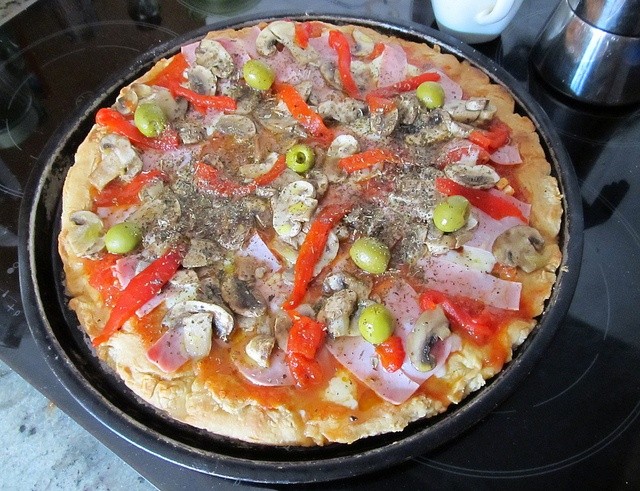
[58,19,567,445]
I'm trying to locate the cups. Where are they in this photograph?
[430,1,523,45]
